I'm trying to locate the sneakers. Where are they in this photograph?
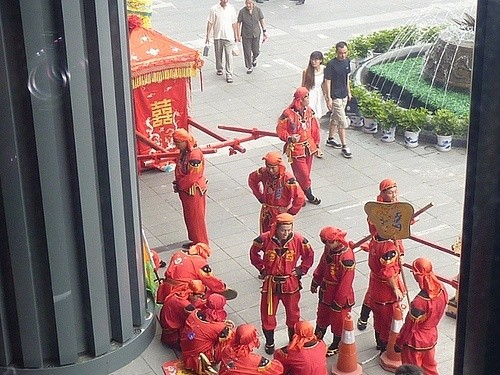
[342,146,352,157]
[326,139,342,148]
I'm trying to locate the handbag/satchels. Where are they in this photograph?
[204,46,209,56]
[345,96,361,117]
[232,42,239,57]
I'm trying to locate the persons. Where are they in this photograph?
[357,179,420,331]
[180,294,237,374]
[156,243,237,304]
[396,363,424,375]
[394,257,447,375]
[150,249,166,271]
[290,0,306,5]
[160,280,205,349]
[276,87,320,205]
[301,51,331,158]
[250,213,314,355]
[273,321,329,375]
[172,128,209,256]
[238,0,268,74]
[311,225,355,357]
[248,151,305,279]
[204,0,239,82]
[325,42,352,159]
[368,234,407,357]
[215,323,283,375]
[256,0,270,4]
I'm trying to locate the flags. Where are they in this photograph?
[142,230,162,310]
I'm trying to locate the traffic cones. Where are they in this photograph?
[331,311,363,375]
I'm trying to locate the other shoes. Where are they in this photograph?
[252,60,256,67]
[357,318,367,331]
[247,68,253,74]
[326,345,339,357]
[308,195,322,204]
[182,243,195,252]
[159,261,166,268]
[226,289,237,300]
[217,70,223,75]
[376,343,381,350]
[226,74,233,83]
[265,343,275,354]
[315,149,324,157]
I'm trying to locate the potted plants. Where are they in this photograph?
[346,85,462,152]
